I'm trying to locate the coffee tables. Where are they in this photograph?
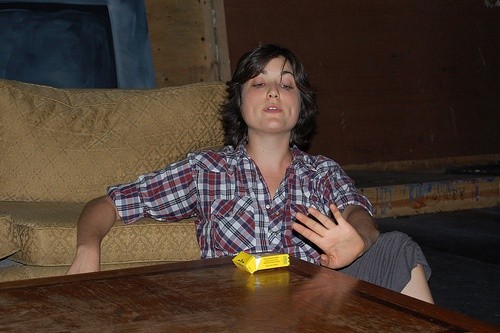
[0,248,500,333]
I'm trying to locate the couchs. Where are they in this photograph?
[1,78,229,282]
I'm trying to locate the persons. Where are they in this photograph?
[66,44,435,306]
